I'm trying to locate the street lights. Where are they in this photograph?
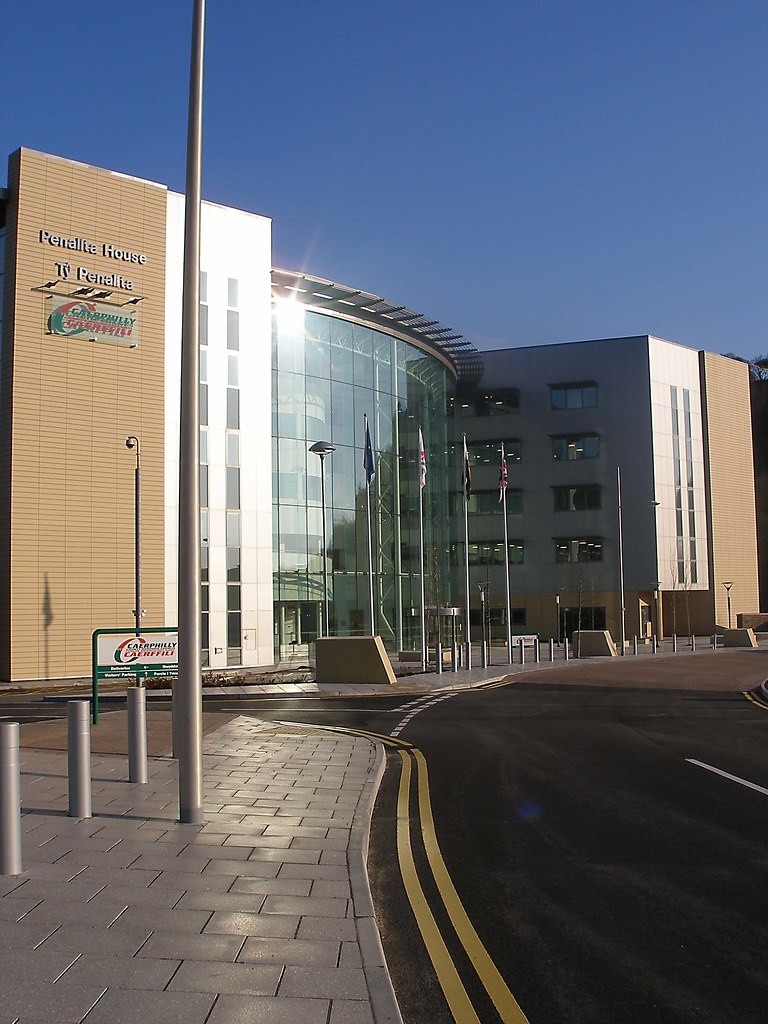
[552,586,564,648]
[125,434,145,687]
[308,439,337,639]
[476,581,491,670]
[649,582,662,647]
[721,581,734,630]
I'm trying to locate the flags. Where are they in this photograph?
[498,451,508,503]
[364,420,375,484]
[461,447,471,501]
[418,428,427,488]
